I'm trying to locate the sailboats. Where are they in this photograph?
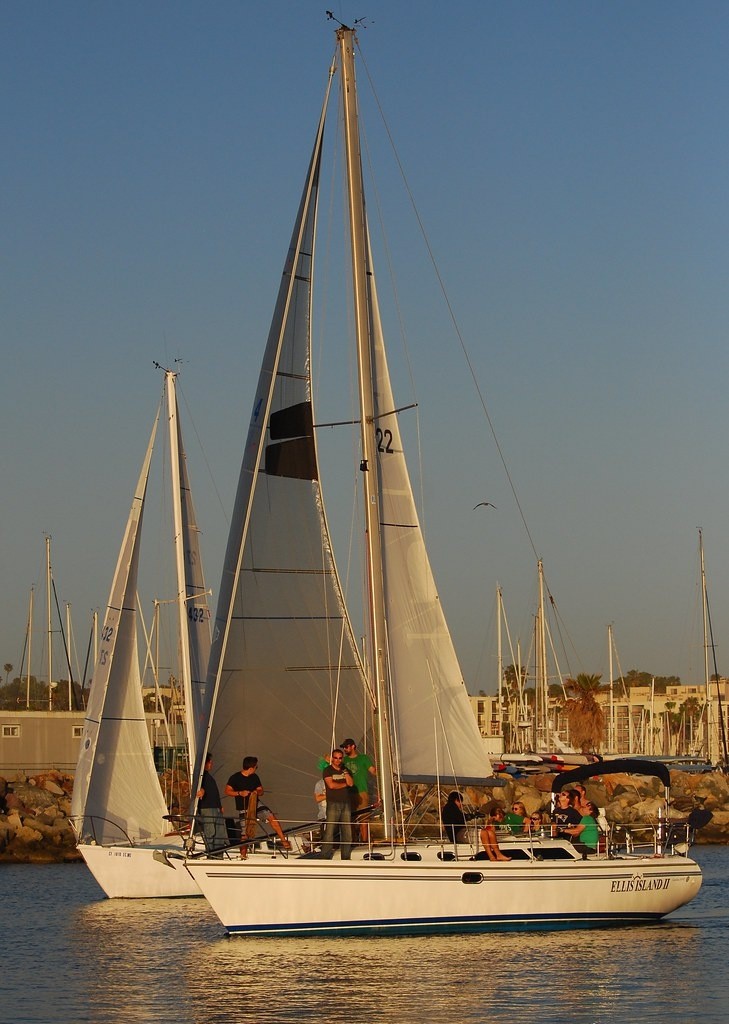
[0,530,190,778]
[481,527,728,777]
[68,360,315,900]
[159,9,708,939]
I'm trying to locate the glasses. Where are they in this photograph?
[333,757,343,760]
[561,793,566,796]
[343,746,348,749]
[512,807,518,811]
[530,817,539,820]
[586,804,590,807]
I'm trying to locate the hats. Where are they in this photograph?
[340,738,355,748]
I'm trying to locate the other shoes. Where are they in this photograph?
[281,840,291,850]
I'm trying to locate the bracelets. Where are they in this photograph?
[238,792,240,795]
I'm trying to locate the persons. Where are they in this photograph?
[314,738,376,860]
[196,752,230,851]
[441,785,600,861]
[224,757,290,859]
